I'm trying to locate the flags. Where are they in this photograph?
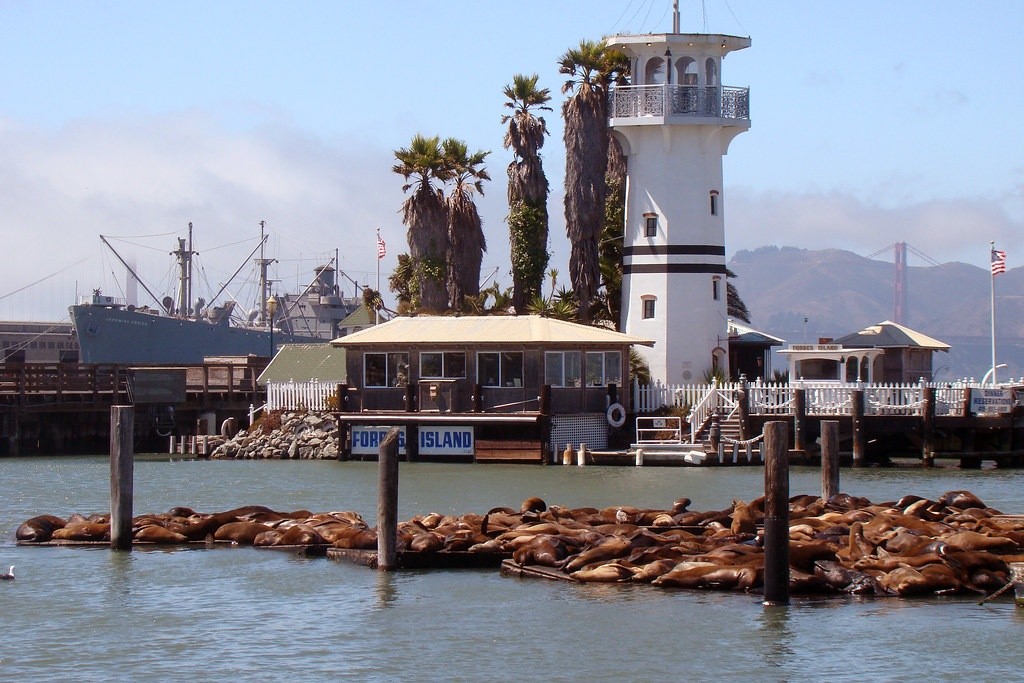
[991,250,1007,276]
[378,235,386,261]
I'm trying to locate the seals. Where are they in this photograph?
[16,490,1024,599]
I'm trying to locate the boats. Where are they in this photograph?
[68,219,391,366]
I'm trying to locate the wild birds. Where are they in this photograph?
[0,566,16,580]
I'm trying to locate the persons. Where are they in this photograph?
[756,356,763,376]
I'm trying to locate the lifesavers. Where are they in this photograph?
[606,403,627,428]
